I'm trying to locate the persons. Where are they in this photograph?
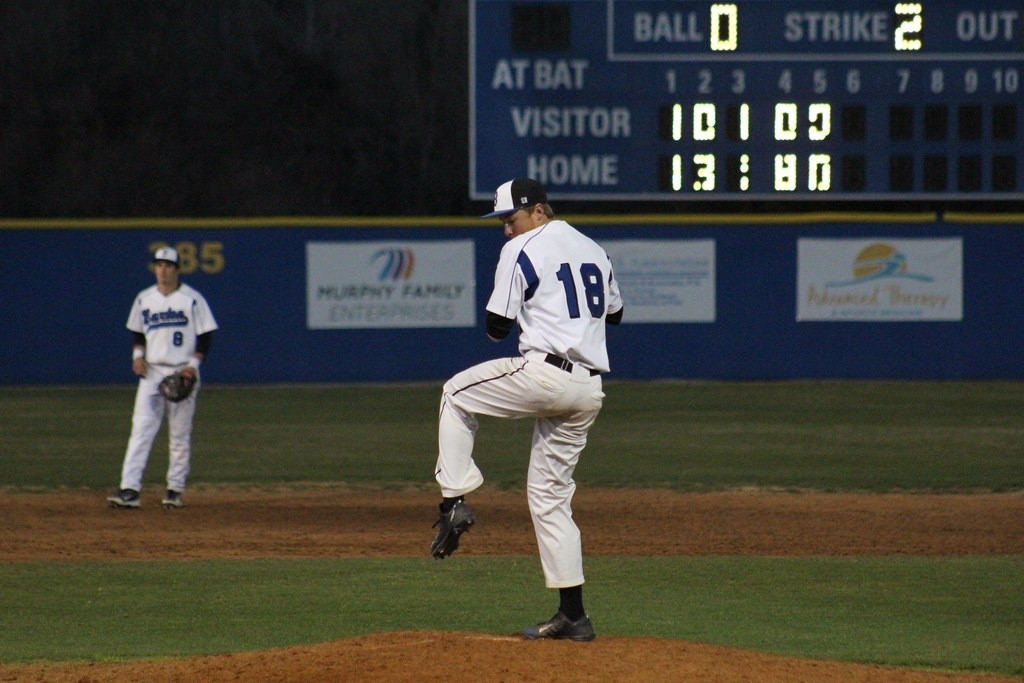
[107,246,218,509]
[429,180,623,643]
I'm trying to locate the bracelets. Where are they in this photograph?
[188,358,200,369]
[133,350,145,359]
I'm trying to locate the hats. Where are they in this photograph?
[152,246,181,269]
[477,177,547,219]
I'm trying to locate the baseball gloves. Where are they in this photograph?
[158,372,196,402]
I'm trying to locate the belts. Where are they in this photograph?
[544,353,600,377]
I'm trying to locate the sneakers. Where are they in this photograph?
[161,490,183,509]
[106,491,141,509]
[430,500,475,560]
[522,612,596,641]
